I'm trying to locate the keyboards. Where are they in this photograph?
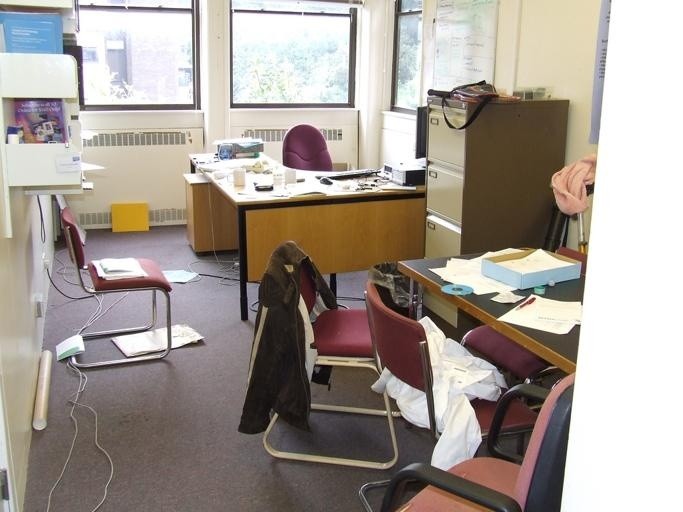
[316,169,382,179]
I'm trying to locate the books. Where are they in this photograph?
[0,12,63,54]
[14,99,66,144]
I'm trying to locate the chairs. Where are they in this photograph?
[60,206,174,371]
[555,246,588,276]
[358,261,579,511]
[281,124,333,176]
[246,241,402,470]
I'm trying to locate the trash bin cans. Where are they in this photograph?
[103,30,132,85]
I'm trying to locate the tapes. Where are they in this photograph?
[534,285,546,295]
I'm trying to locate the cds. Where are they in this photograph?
[441,284,474,296]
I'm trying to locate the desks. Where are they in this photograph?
[183,151,428,320]
[396,243,584,406]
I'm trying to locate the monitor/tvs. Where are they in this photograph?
[415,106,428,167]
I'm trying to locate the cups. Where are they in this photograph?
[272,168,297,197]
[227,168,246,185]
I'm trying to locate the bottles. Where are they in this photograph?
[67,115,83,153]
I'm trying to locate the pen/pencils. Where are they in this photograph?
[519,297,535,308]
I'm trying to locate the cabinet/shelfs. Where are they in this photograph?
[420,99,569,329]
[1,51,88,238]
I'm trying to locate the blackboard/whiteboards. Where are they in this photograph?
[432,0,498,92]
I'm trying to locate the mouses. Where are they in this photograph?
[320,177,332,185]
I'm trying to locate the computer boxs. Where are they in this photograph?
[384,159,425,186]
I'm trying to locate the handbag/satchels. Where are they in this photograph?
[441,80,499,131]
